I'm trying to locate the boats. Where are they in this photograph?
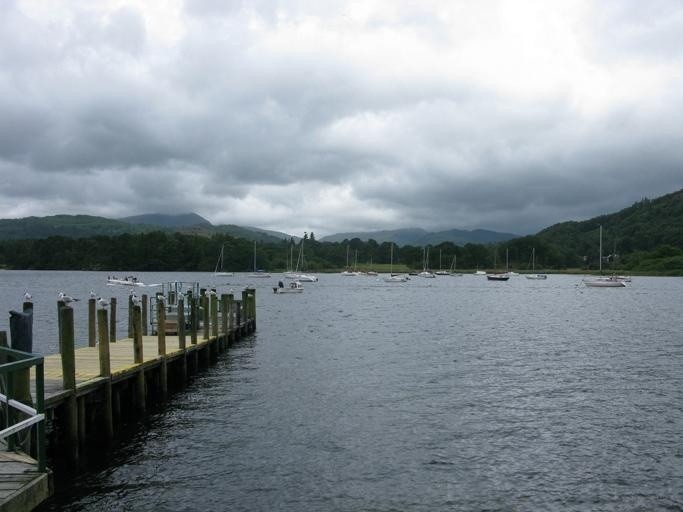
[107,276,146,289]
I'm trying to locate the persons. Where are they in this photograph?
[279,280,284,289]
[108,275,137,283]
